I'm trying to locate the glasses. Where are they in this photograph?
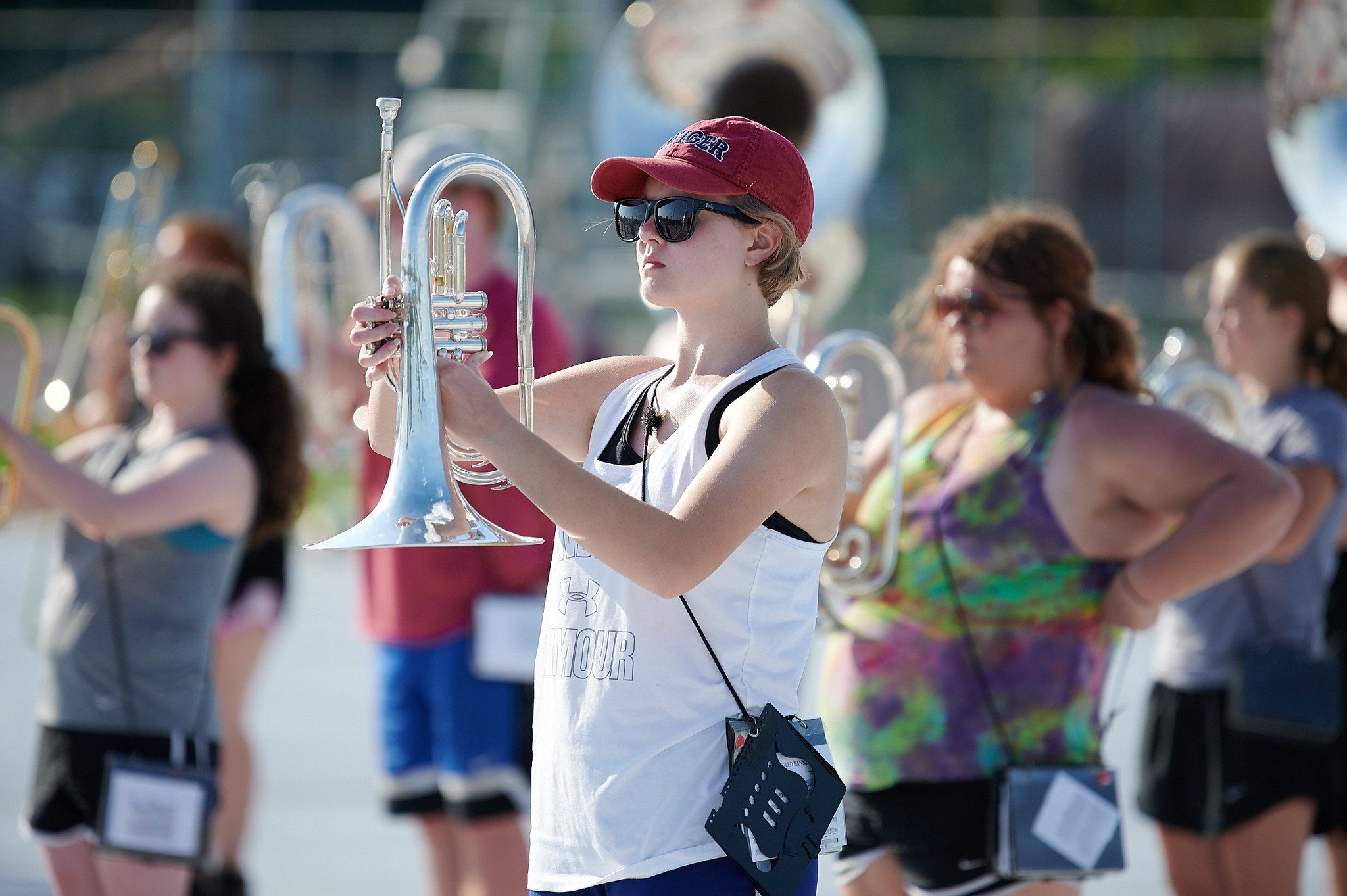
[611,194,761,243]
[923,291,1009,327]
[125,327,208,354]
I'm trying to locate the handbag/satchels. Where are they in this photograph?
[1227,632,1340,740]
[990,765,1129,880]
[98,752,218,858]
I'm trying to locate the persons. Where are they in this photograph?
[817,208,1347,895]
[0,212,309,896]
[335,55,847,896]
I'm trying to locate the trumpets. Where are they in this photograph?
[1140,333,1241,440]
[304,96,545,553]
[2,304,35,525]
[233,163,373,368]
[783,290,907,588]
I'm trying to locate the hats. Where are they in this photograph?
[589,114,815,247]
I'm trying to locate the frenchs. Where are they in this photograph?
[593,0,886,359]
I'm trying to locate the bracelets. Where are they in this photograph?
[1118,567,1161,609]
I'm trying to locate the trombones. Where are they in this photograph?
[38,135,179,440]
